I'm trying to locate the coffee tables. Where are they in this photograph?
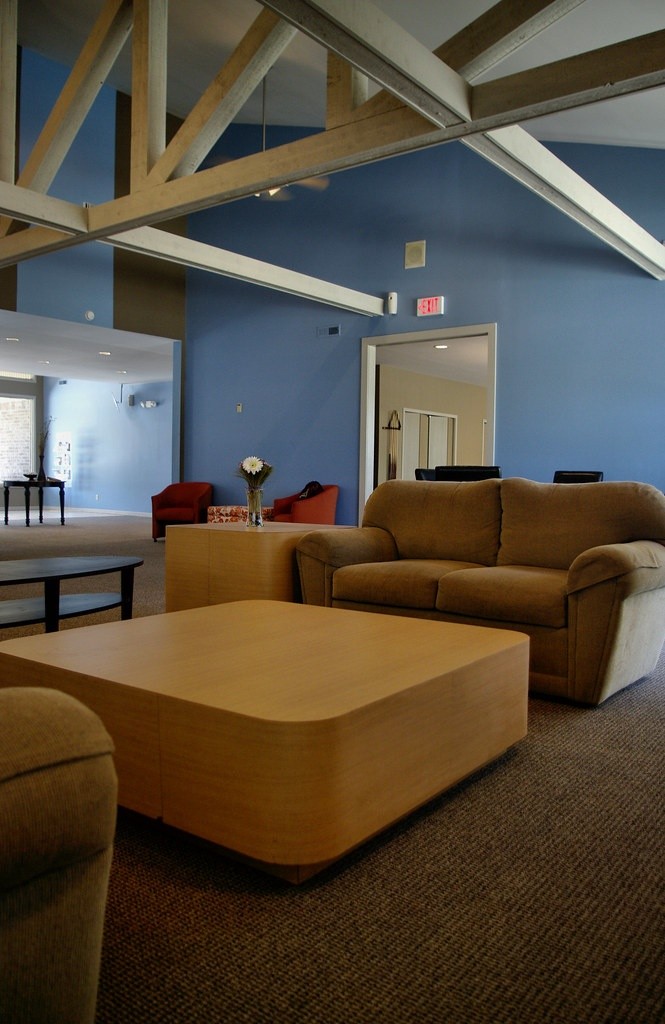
[0,598,532,886]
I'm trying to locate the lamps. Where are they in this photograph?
[140,400,157,408]
[433,340,448,349]
[253,188,281,197]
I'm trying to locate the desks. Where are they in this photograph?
[206,505,274,525]
[164,521,357,608]
[3,480,66,527]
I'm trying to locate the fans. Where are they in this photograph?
[259,66,330,202]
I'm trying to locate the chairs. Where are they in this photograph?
[152,482,214,542]
[272,485,340,525]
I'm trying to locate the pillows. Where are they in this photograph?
[297,480,324,499]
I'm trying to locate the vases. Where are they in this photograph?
[244,486,264,527]
[37,456,47,481]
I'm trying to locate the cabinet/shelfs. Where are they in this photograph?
[0,555,145,633]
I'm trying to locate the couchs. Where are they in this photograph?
[0,686,120,1024]
[295,478,665,709]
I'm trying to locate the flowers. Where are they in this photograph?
[232,456,273,526]
